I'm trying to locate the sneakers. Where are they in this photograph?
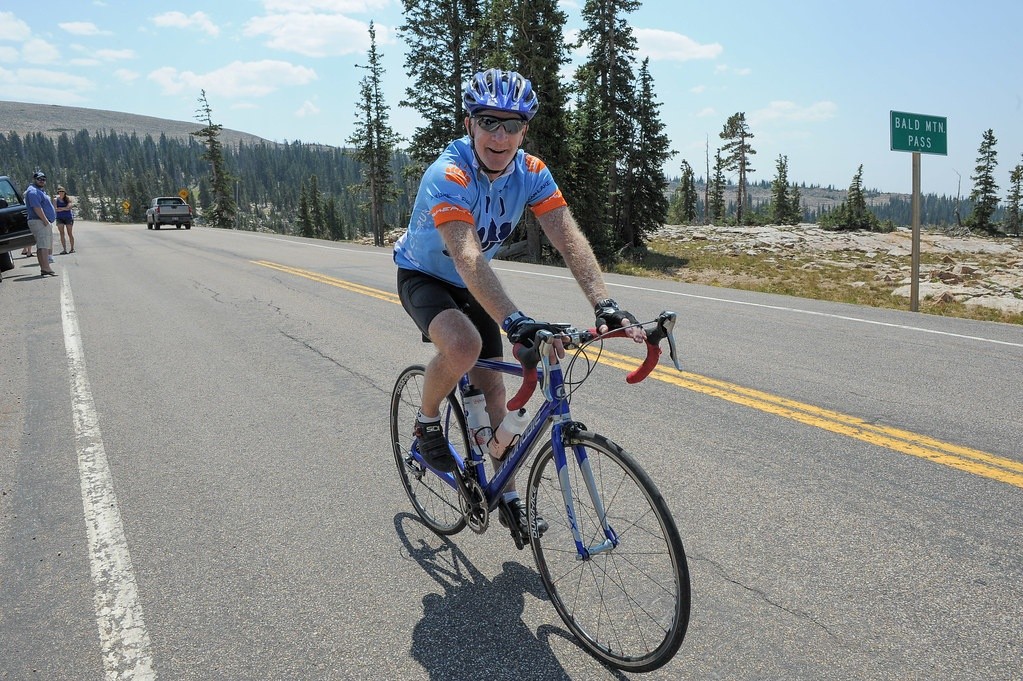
[499,498,549,536]
[412,417,457,472]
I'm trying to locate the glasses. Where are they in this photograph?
[472,115,528,134]
[59,191,64,192]
[37,177,46,181]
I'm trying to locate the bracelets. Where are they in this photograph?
[594,298,614,312]
[501,309,524,331]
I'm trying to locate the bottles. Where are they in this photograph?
[487,408,531,459]
[463,384,493,455]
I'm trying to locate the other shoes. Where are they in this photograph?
[69,250,75,253]
[49,259,55,262]
[60,252,67,254]
[41,270,58,277]
[27,254,34,257]
[22,252,28,254]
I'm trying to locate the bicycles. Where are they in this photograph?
[388,308,693,675]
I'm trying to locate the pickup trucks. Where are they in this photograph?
[144,196,192,230]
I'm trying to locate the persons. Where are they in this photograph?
[25,172,59,278]
[22,246,34,257]
[53,187,76,254]
[393,68,647,538]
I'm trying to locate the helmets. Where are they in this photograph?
[463,68,539,121]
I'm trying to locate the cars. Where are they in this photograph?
[0,176,37,282]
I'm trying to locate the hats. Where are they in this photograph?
[34,172,46,178]
[57,187,65,192]
